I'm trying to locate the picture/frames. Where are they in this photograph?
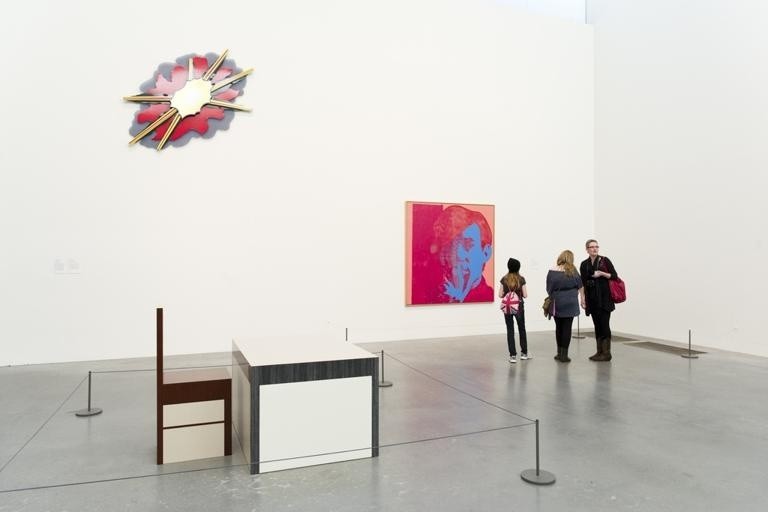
[404,201,495,305]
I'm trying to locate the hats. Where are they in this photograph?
[506,257,521,273]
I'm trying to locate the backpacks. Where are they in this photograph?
[500,279,521,316]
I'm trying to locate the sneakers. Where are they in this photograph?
[519,351,534,360]
[508,355,517,363]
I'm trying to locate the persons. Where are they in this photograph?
[579,239,619,363]
[546,249,587,363]
[498,257,534,364]
[430,204,494,304]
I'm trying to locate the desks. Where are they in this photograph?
[232,336,379,475]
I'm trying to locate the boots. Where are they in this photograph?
[553,344,560,360]
[588,335,613,361]
[559,346,572,362]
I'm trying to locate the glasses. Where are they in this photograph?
[586,245,599,249]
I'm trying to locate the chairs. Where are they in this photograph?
[156,308,233,465]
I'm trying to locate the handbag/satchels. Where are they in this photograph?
[541,294,554,320]
[600,256,628,303]
